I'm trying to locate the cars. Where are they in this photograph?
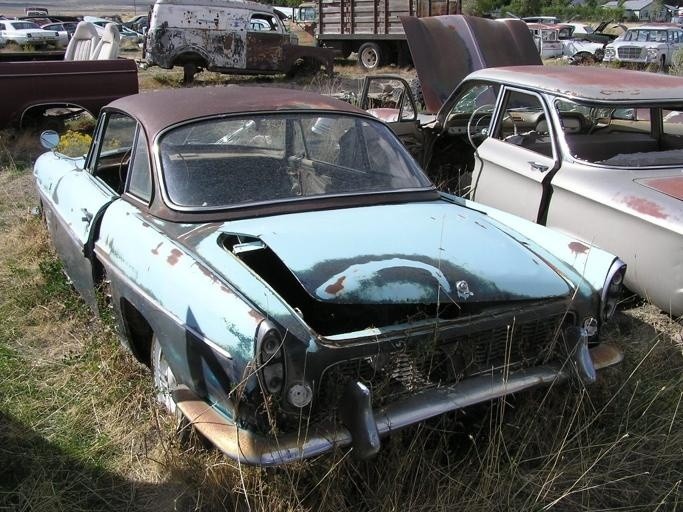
[24,82,636,470]
[312,13,682,327]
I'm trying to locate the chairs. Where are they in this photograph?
[91,23,121,60]
[64,21,100,61]
